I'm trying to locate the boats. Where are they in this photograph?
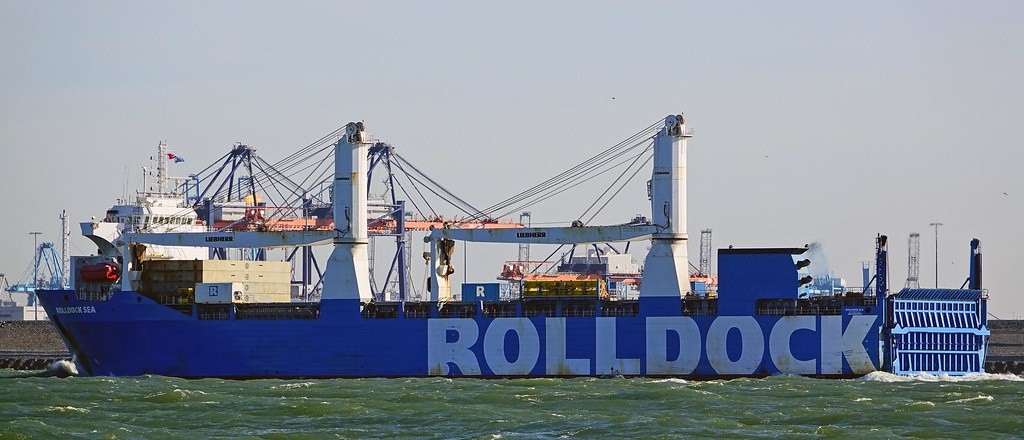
[47,114,992,380]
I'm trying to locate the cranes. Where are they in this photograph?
[699,228,712,278]
[903,231,923,289]
[0,241,70,307]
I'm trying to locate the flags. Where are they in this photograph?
[168,153,185,163]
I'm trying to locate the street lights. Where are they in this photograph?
[930,221,944,288]
[29,230,43,321]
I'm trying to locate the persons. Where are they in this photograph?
[440,304,448,314]
[463,303,475,316]
